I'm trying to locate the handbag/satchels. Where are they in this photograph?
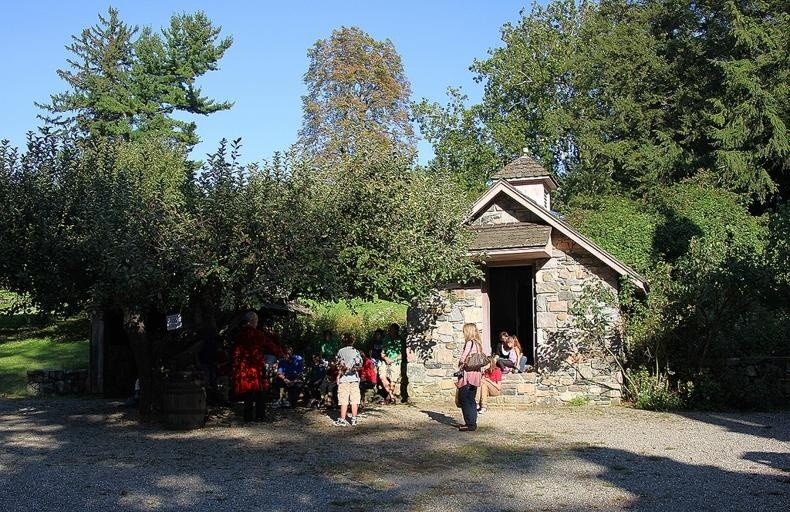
[463,350,490,372]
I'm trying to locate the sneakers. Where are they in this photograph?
[332,418,347,428]
[350,416,359,426]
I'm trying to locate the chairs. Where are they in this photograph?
[296,366,327,410]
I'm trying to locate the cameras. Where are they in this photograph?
[454,369,464,379]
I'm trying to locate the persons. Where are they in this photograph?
[454,323,483,432]
[474,331,527,414]
[265,323,407,427]
[230,311,284,424]
[125,379,142,406]
[215,346,230,401]
